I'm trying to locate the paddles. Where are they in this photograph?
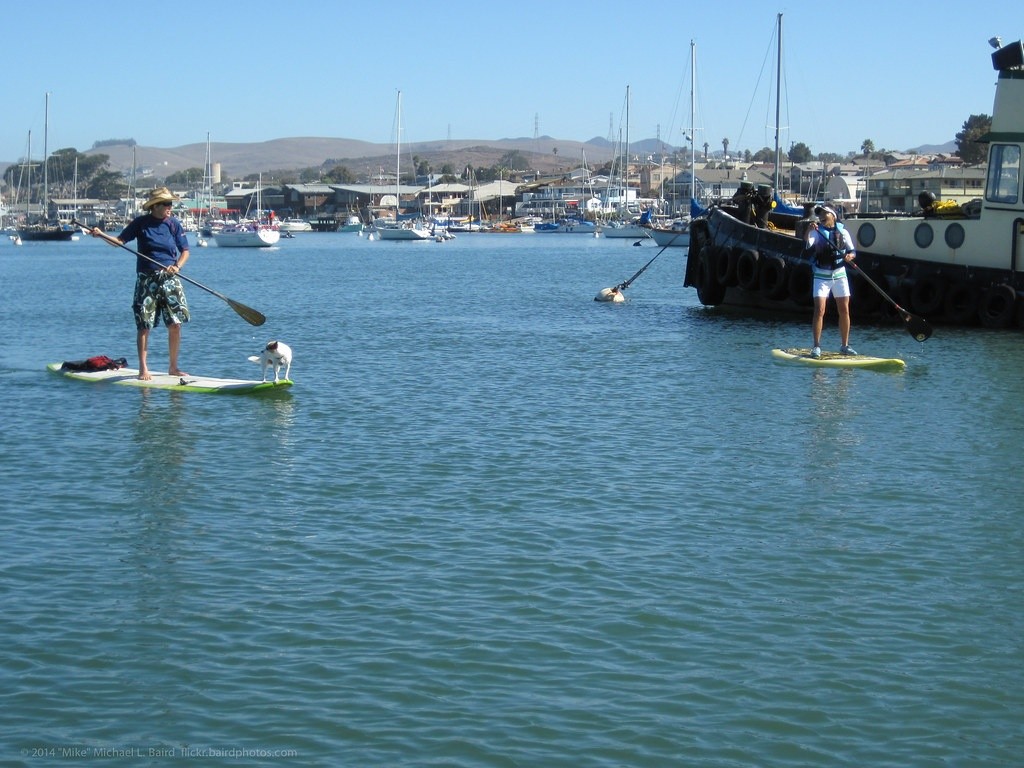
[69,220,267,325]
[813,222,935,343]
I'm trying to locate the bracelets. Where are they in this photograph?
[175,264,181,270]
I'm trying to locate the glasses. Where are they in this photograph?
[156,202,173,206]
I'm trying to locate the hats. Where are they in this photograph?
[143,187,178,211]
[814,207,837,222]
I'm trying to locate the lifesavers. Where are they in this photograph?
[910,275,944,315]
[759,259,787,301]
[737,248,760,290]
[852,267,886,306]
[788,263,813,304]
[941,265,976,296]
[978,284,1017,327]
[695,251,723,304]
[881,283,912,322]
[715,248,736,284]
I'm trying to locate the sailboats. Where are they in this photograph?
[428,11,806,248]
[0,92,225,241]
[371,91,451,240]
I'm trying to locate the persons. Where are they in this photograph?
[803,204,856,359]
[85,187,191,381]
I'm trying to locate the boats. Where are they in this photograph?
[212,173,281,247]
[683,38,1024,330]
[281,217,312,232]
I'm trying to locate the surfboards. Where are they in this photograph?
[45,362,293,395]
[770,347,907,370]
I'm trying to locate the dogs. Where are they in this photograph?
[247,341,292,381]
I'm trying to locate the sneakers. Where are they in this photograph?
[811,347,821,359]
[840,346,857,355]
